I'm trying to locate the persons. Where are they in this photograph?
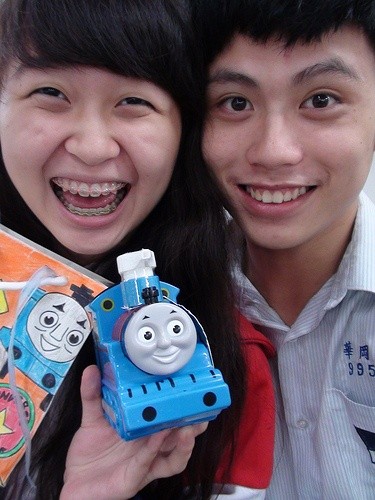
[174,0,374,498]
[0,0,277,499]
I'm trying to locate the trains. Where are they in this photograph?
[85,249,231,441]
